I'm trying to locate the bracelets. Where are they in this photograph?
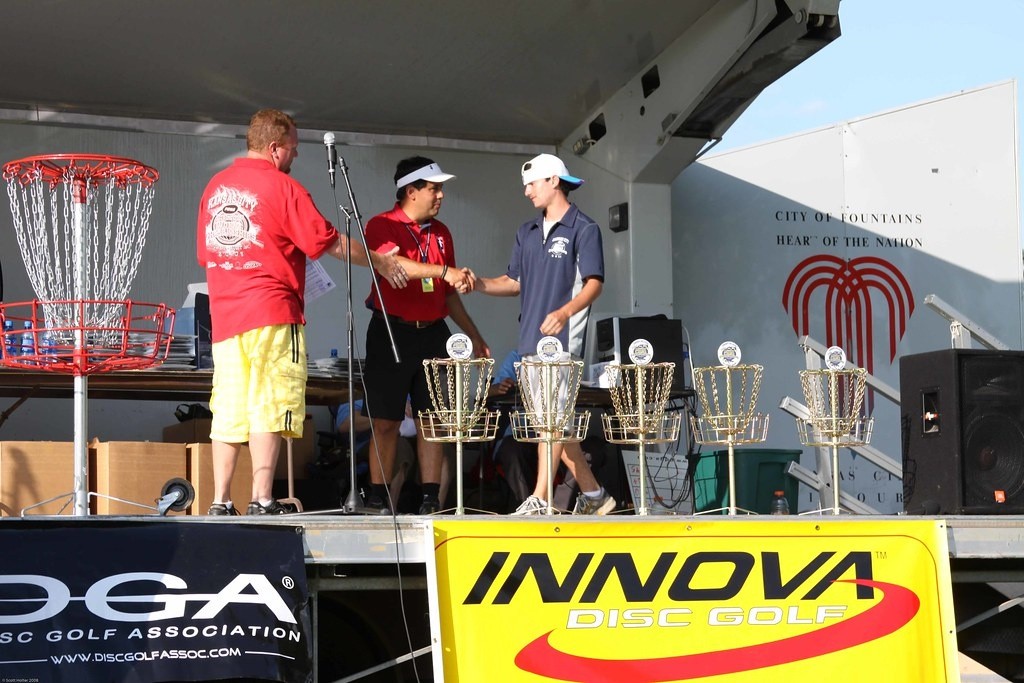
[440,264,447,279]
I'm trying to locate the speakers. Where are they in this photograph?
[899,349,1024,515]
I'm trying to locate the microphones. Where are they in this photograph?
[324,133,336,187]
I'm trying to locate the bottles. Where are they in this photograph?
[19,320,35,366]
[0,320,18,364]
[329,349,338,358]
[40,320,58,365]
[771,491,788,515]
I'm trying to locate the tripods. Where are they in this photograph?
[278,154,402,517]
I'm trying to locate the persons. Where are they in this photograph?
[364,156,491,515]
[334,392,455,516]
[196,108,410,516]
[487,312,591,514]
[449,153,617,517]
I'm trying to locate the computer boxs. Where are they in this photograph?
[595,317,685,393]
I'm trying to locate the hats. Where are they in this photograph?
[521,153,585,191]
[397,162,457,188]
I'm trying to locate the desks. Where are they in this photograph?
[478,384,692,511]
[0,363,367,512]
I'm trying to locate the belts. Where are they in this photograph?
[376,311,439,328]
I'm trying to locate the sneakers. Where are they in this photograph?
[208,502,241,516]
[420,503,440,517]
[511,495,548,515]
[246,498,293,515]
[571,487,617,515]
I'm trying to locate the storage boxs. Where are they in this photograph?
[687,448,802,518]
[186,442,253,517]
[89,437,186,518]
[1,441,88,518]
